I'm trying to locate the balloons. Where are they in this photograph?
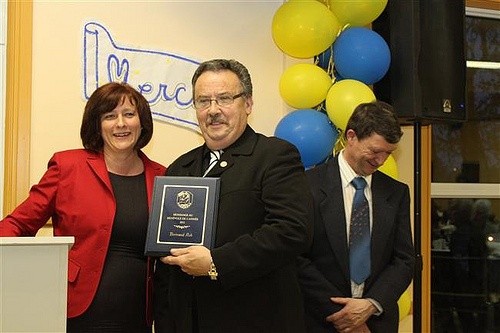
[271,0,398,181]
[397,287,411,320]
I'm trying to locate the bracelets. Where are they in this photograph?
[207,257,218,281]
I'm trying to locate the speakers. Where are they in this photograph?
[372,0,467,127]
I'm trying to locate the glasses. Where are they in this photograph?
[193,92,244,110]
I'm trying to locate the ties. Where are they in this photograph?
[349,177,371,285]
[202,150,224,178]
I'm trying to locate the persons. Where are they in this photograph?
[0,82,167,333]
[148,58,314,333]
[290,100,416,333]
